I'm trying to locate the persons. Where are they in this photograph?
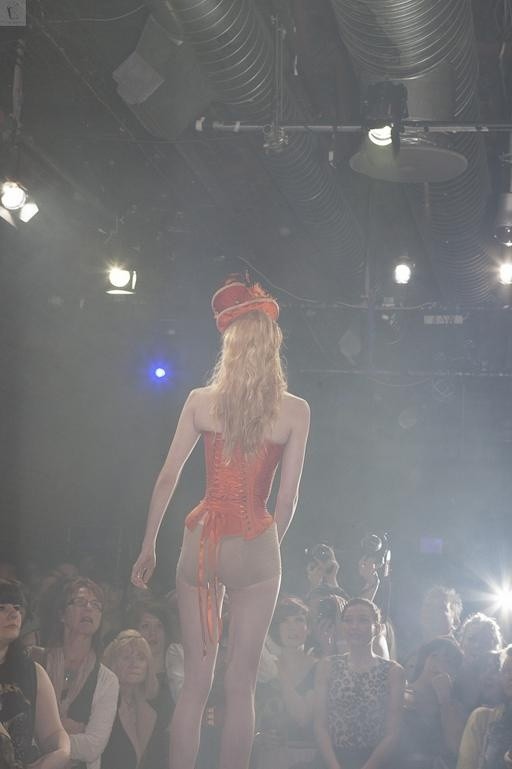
[129,279,311,769]
[3,544,510,767]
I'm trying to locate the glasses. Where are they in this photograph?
[68,598,104,613]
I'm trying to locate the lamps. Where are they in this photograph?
[0,177,39,228]
[363,80,406,155]
[103,266,137,295]
[390,255,416,286]
[496,257,511,298]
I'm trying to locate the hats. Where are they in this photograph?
[208,280,282,333]
